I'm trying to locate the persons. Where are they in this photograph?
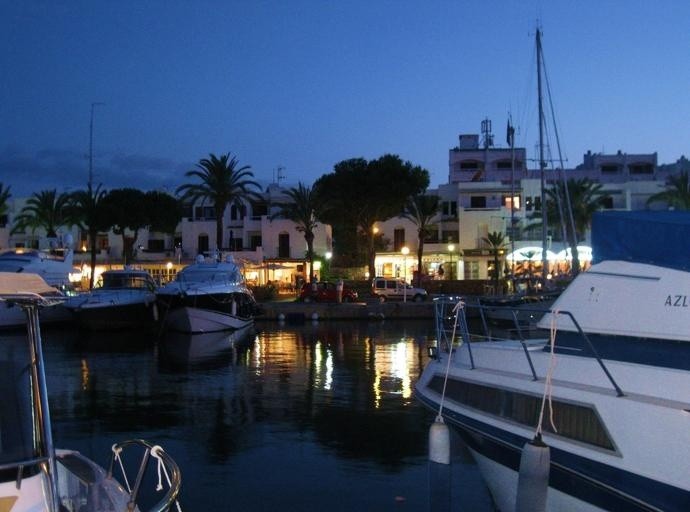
[335,276,344,304]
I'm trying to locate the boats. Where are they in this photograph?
[0,246,257,340]
[1,269,183,512]
[414,201,688,509]
[475,21,585,326]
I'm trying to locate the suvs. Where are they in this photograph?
[372,275,427,301]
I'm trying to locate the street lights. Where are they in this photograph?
[448,243,455,281]
[401,247,410,303]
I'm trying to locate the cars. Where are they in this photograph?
[300,280,359,303]
